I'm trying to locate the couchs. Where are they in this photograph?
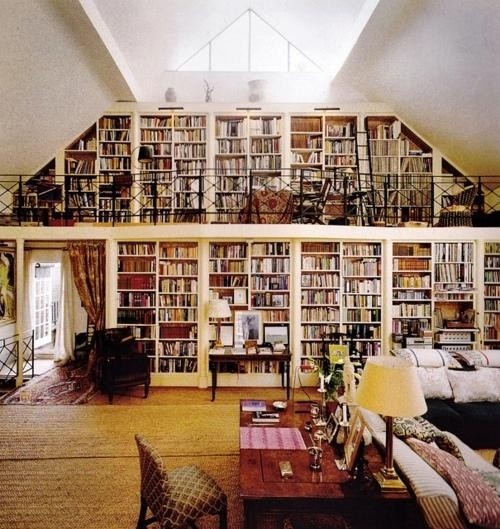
[384,348,500,451]
[334,394,500,529]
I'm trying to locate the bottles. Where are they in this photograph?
[315,417,325,424]
[305,421,312,432]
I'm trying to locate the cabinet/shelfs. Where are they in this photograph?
[209,241,293,373]
[392,240,478,350]
[301,241,383,374]
[13,114,486,221]
[118,240,199,375]
[484,240,500,348]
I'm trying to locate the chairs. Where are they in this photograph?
[318,331,364,401]
[97,325,152,407]
[132,432,228,529]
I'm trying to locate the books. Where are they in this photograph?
[241,400,266,411]
[240,426,307,450]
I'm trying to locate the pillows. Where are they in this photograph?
[392,367,500,529]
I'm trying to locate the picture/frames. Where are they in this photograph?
[0,246,18,326]
[345,407,367,470]
[324,414,339,444]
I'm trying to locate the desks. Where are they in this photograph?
[207,348,292,402]
[237,398,415,529]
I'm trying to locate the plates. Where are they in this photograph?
[272,401,287,409]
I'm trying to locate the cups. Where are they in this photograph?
[308,447,323,469]
[311,404,318,417]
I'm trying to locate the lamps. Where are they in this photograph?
[352,355,428,494]
